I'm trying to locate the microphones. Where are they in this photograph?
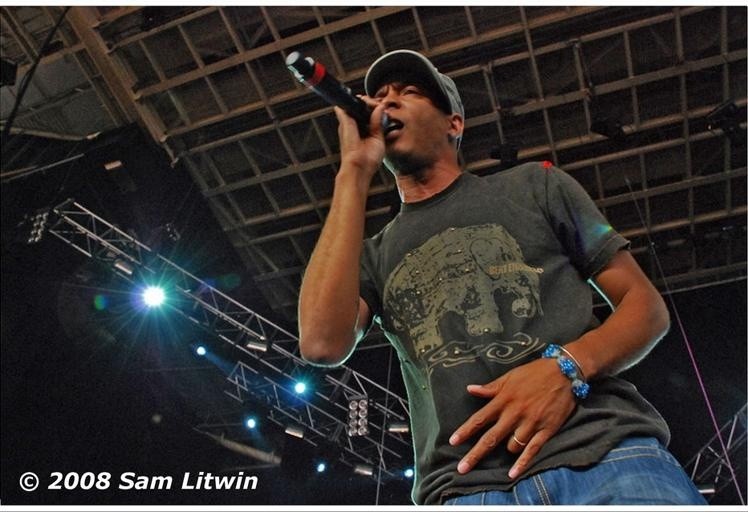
[285,52,392,137]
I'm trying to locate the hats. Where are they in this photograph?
[365,48,465,150]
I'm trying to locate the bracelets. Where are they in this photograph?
[543,344,589,404]
[559,346,586,379]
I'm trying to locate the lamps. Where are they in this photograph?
[683,223,703,248]
[706,101,746,147]
[579,48,632,145]
[649,230,668,255]
[720,216,747,239]
[489,143,520,170]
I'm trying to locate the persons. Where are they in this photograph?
[295,48,710,504]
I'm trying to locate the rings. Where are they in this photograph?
[512,437,526,446]
